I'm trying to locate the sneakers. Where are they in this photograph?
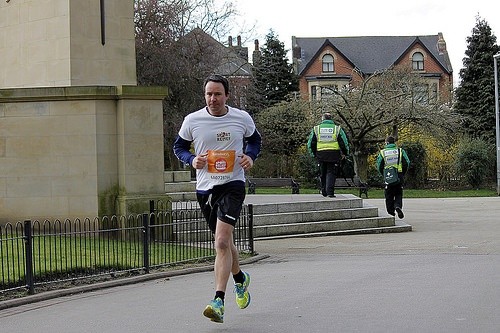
[232,270,250,309]
[203,298,224,322]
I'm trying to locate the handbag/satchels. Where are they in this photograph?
[382,167,400,184]
[336,155,356,178]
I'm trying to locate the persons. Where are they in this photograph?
[308,113,349,199]
[172,76,262,324]
[376,137,410,221]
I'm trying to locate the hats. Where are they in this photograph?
[321,113,331,120]
[385,137,395,144]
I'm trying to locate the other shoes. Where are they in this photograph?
[391,212,396,216]
[323,193,326,196]
[329,196,336,198]
[395,208,404,219]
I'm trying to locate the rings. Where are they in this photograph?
[244,164,247,168]
